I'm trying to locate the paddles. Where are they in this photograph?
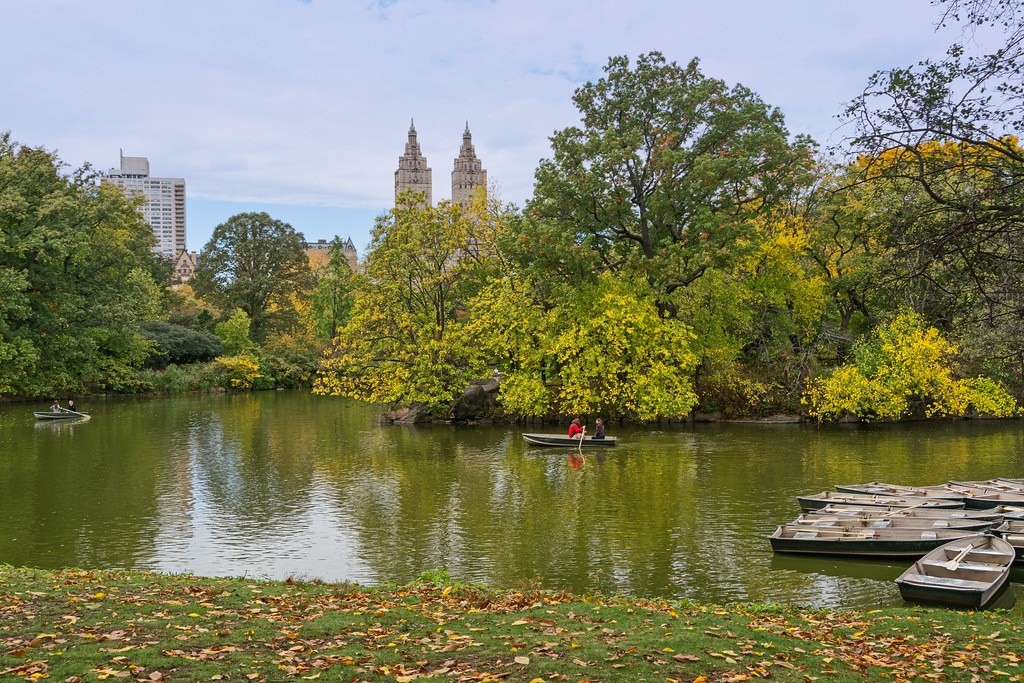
[59,407,90,418]
[945,543,974,571]
[578,425,586,450]
[788,497,928,539]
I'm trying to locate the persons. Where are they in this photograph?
[568,418,588,440]
[591,418,606,439]
[49,400,62,413]
[68,400,76,412]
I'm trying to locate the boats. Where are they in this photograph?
[34,412,89,419]
[767,478,1024,555]
[520,433,616,446]
[897,534,1016,610]
